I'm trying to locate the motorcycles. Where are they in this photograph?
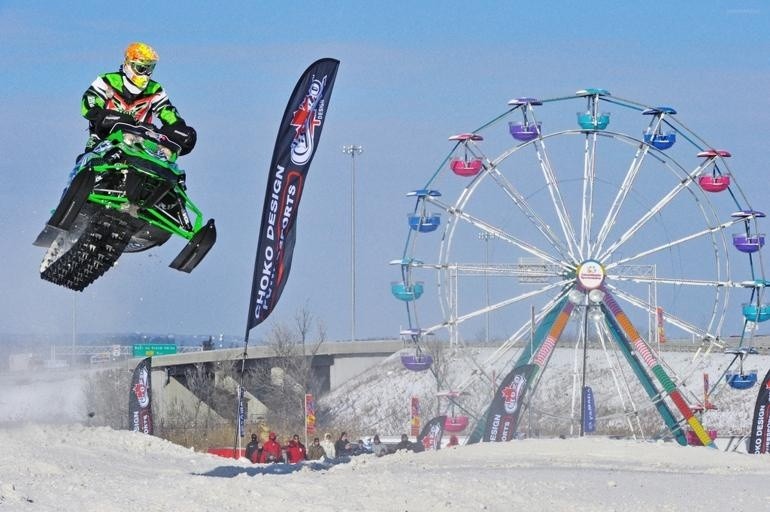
[29,109,217,299]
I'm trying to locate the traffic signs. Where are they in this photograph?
[133,343,177,359]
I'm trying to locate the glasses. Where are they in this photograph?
[134,65,152,75]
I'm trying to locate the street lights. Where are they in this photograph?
[339,142,363,340]
[477,230,496,341]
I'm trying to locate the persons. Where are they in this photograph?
[65,39,188,212]
[240,428,459,464]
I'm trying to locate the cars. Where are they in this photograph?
[724,347,758,355]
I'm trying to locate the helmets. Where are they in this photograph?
[122,42,159,89]
